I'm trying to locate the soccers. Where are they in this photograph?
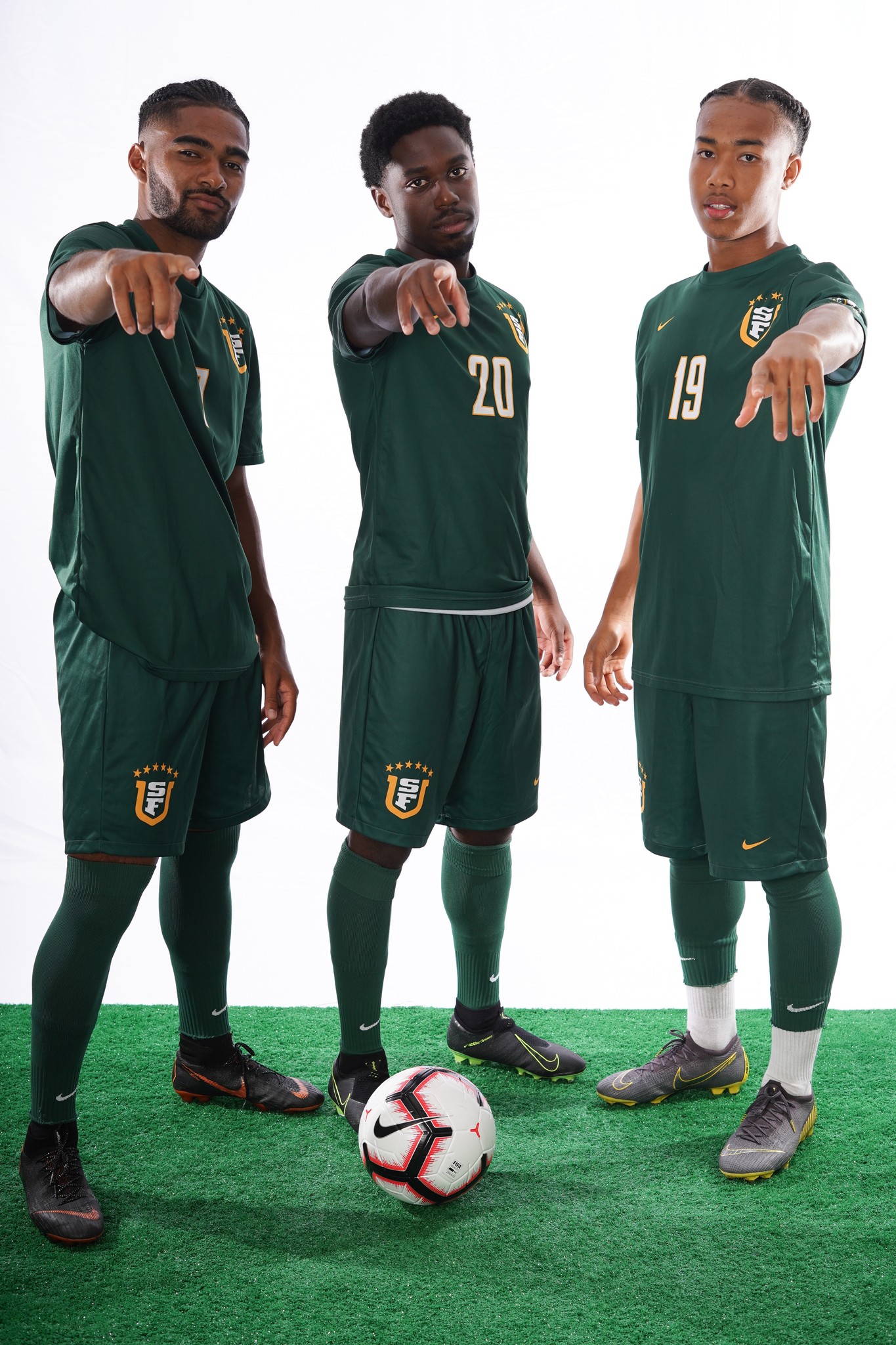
[357,1066,496,1207]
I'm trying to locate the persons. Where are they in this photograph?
[15,78,326,1250]
[327,93,587,1135]
[583,78,867,1182]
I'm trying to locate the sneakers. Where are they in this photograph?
[327,1048,390,1134]
[171,1042,325,1113]
[597,1029,749,1106]
[719,1079,817,1181]
[446,1006,587,1084]
[19,1127,105,1244]
